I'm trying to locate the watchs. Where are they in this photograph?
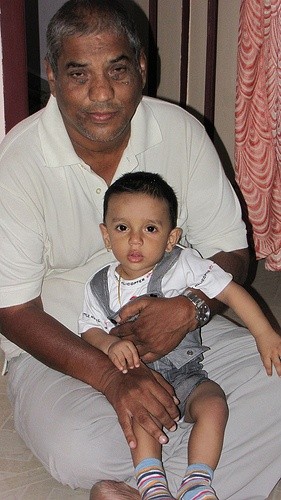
[182,291,210,326]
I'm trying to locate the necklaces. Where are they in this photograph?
[118,270,122,308]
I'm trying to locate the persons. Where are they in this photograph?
[0,0,281,500]
[78,171,281,500]
